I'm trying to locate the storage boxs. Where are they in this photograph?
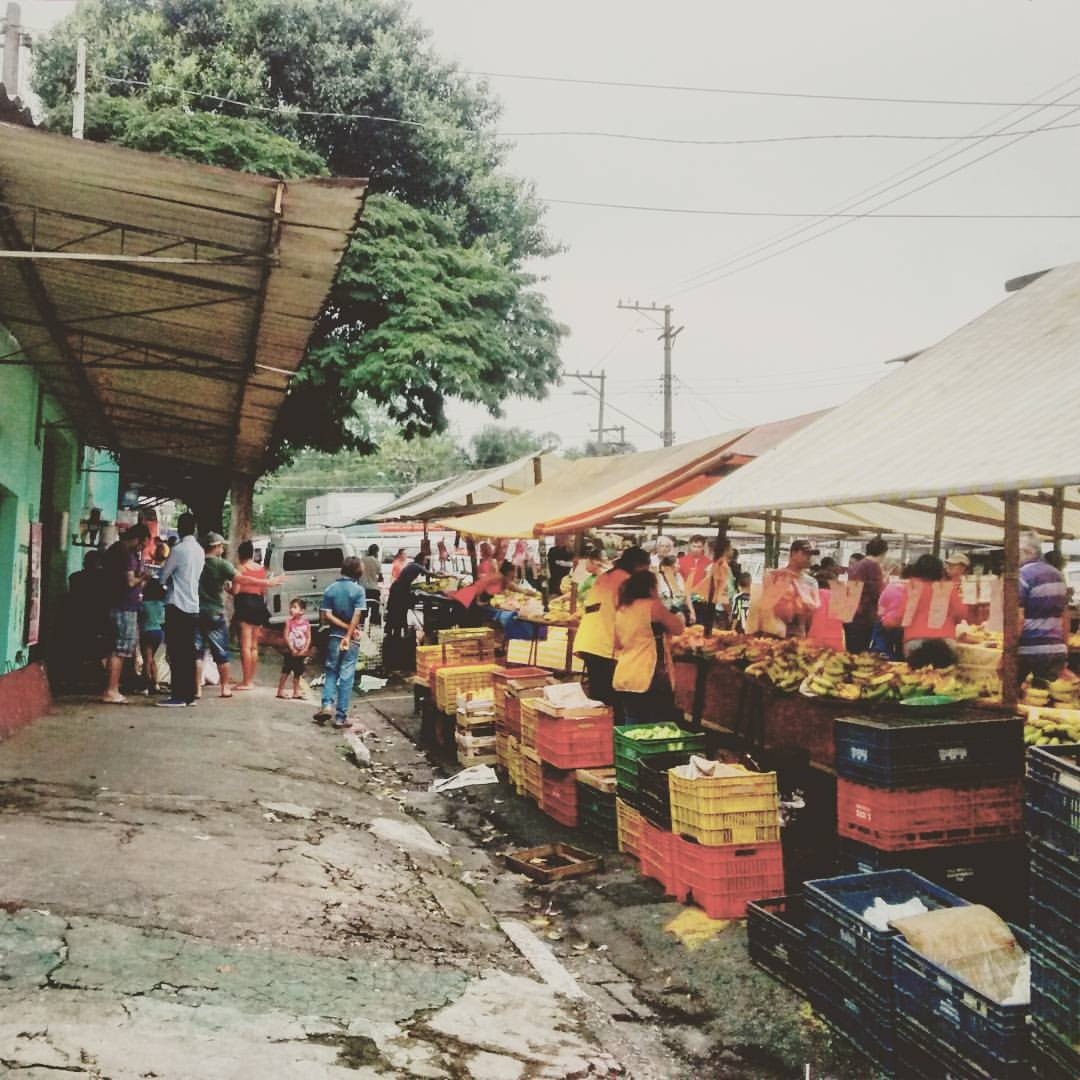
[413,627,1080,1080]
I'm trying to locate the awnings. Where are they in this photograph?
[0,123,370,477]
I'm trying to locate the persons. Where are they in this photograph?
[478,530,1068,726]
[276,599,311,700]
[313,557,368,728]
[66,507,287,707]
[358,537,450,640]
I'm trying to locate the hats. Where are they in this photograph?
[945,553,969,566]
[207,532,230,546]
[139,510,157,521]
[792,540,820,554]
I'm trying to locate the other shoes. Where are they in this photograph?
[187,698,197,706]
[313,709,332,722]
[193,692,201,699]
[276,693,291,699]
[102,689,129,704]
[221,689,232,697]
[231,683,254,691]
[292,693,307,700]
[155,698,186,708]
[334,719,353,729]
[132,683,160,697]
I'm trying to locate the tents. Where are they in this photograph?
[667,260,1080,717]
[356,446,573,536]
[435,406,837,675]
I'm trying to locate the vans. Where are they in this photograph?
[351,538,442,580]
[263,525,354,628]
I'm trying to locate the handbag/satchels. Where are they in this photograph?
[519,577,536,594]
[205,654,220,685]
[774,572,798,625]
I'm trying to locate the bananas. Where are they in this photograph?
[412,579,451,595]
[669,624,1080,746]
[491,593,584,623]
